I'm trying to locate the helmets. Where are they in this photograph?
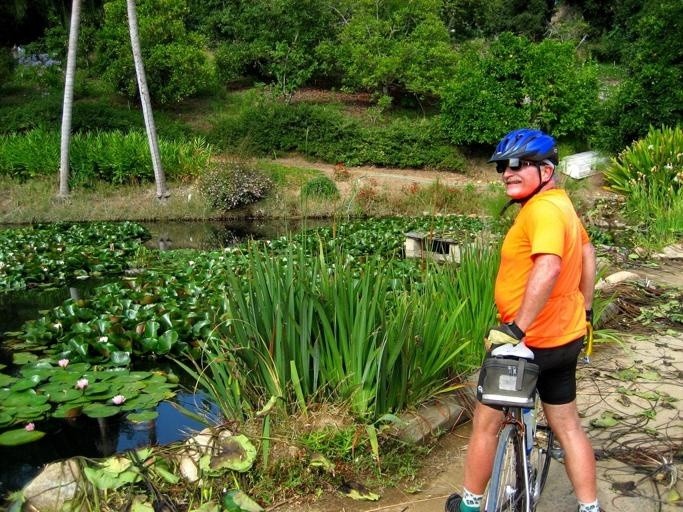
[487,128,559,165]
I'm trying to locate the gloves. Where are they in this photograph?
[483,322,524,351]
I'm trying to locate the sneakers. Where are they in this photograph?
[446,493,462,511]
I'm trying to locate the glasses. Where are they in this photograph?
[495,160,544,173]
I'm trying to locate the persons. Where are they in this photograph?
[444,130,601,512]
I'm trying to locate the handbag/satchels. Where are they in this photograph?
[478,360,539,410]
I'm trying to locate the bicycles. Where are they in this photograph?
[474,312,596,512]
